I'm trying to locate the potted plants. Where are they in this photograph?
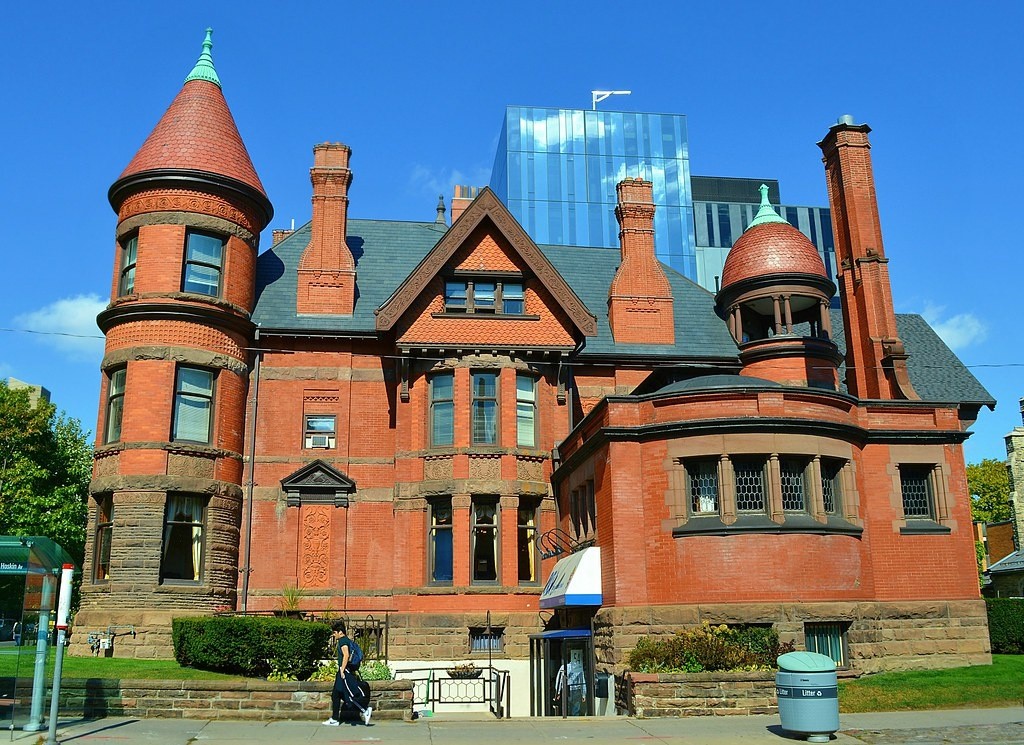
[315,598,342,627]
[273,584,308,620]
[447,663,484,679]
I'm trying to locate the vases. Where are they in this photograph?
[352,625,383,638]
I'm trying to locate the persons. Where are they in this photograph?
[555,653,586,715]
[12,618,35,647]
[321,622,372,727]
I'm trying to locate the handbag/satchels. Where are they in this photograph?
[352,667,370,707]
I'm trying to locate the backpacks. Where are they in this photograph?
[337,636,363,670]
[13,622,22,634]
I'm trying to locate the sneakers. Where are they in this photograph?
[361,705,372,725]
[321,717,339,727]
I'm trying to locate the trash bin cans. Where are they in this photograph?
[773,651,841,744]
